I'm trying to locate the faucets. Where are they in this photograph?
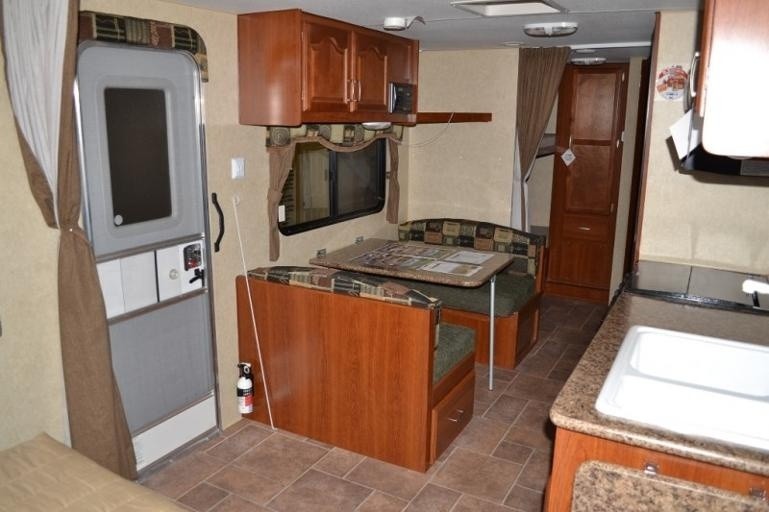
[740,280,768,296]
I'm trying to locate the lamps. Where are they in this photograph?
[523,21,578,38]
[571,57,607,66]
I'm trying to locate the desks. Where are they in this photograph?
[311,238,516,392]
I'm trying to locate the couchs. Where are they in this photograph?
[386,216,548,372]
[234,264,478,474]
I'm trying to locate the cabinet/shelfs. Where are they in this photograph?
[387,42,419,114]
[302,22,390,114]
[687,0,768,119]
[541,62,629,307]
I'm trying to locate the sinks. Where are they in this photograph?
[626,330,769,400]
